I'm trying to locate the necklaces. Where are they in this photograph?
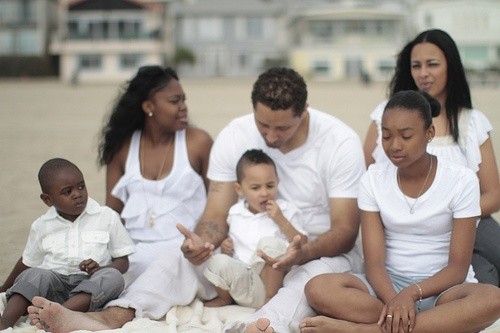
[141,133,174,230]
[398,154,433,214]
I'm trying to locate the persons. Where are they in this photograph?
[198,149,310,309]
[27,67,368,333]
[97,65,214,286]
[299,89,500,333]
[0,158,137,333]
[362,29,500,288]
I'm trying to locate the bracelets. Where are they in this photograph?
[415,283,423,311]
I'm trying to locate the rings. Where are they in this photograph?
[386,314,393,318]
